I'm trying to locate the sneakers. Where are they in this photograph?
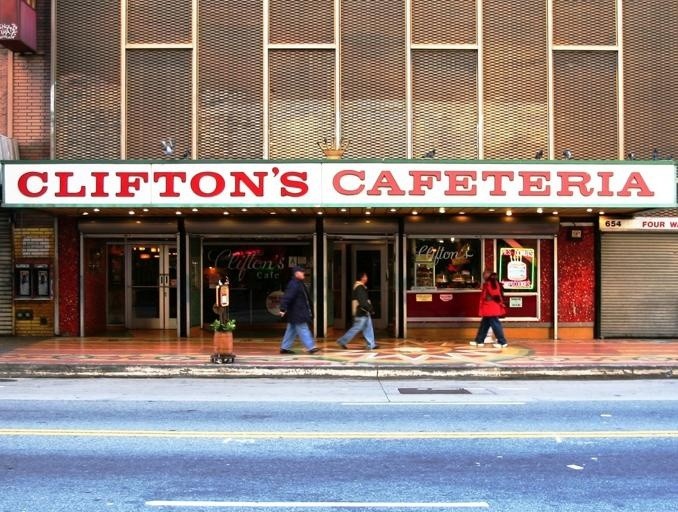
[279,349,294,355]
[493,342,508,348]
[483,336,493,345]
[340,345,347,350]
[373,345,380,349]
[469,341,484,348]
[309,347,320,354]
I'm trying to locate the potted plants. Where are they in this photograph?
[209,318,237,356]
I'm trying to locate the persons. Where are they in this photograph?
[278,266,319,354]
[477,273,507,348]
[469,271,501,348]
[335,272,378,350]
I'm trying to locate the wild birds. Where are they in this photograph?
[626,151,636,160]
[534,149,543,160]
[161,135,174,153]
[660,369,673,379]
[563,149,572,161]
[421,148,436,160]
[651,147,658,160]
[662,155,673,160]
[179,150,191,158]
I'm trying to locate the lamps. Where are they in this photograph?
[158,139,177,160]
[416,145,674,162]
[179,149,192,159]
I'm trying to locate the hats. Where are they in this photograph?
[293,265,305,273]
